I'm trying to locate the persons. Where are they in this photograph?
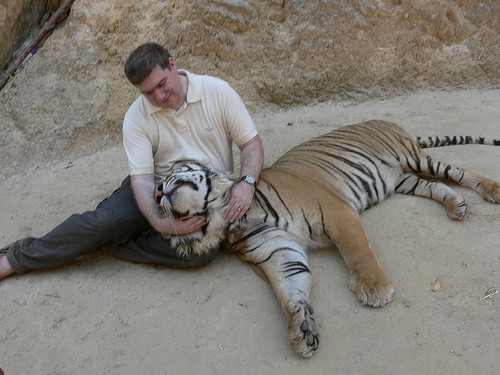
[0,43,264,281]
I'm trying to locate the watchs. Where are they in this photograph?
[241,175,256,186]
